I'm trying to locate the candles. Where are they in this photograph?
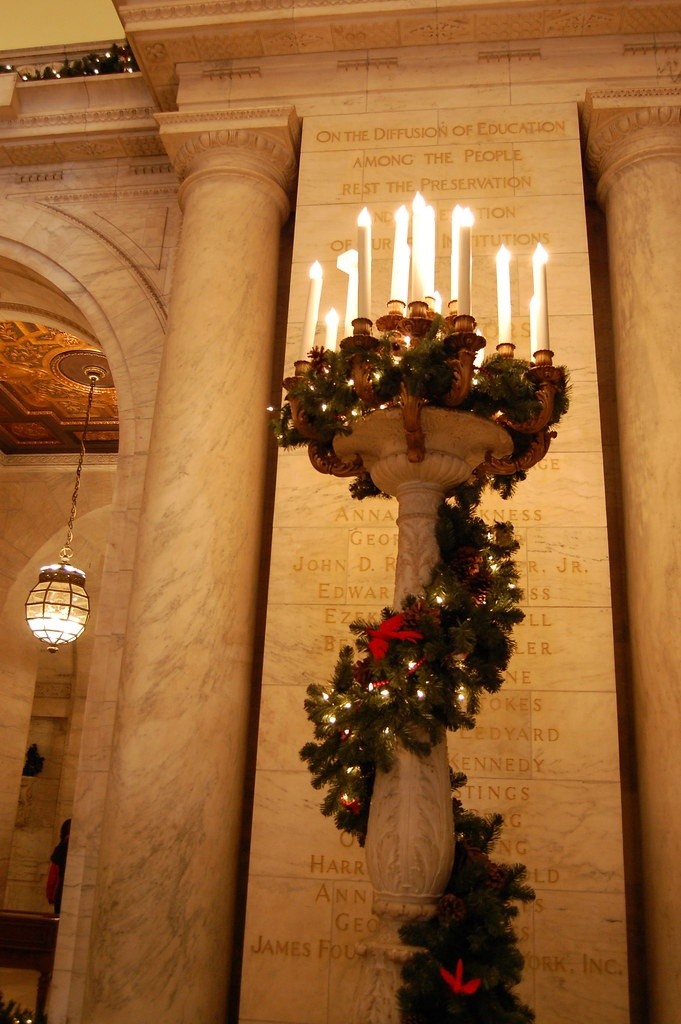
[295,190,549,386]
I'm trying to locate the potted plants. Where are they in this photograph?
[10,742,45,828]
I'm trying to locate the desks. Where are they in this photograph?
[0,909,60,1024]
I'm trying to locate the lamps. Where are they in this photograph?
[23,379,97,654]
